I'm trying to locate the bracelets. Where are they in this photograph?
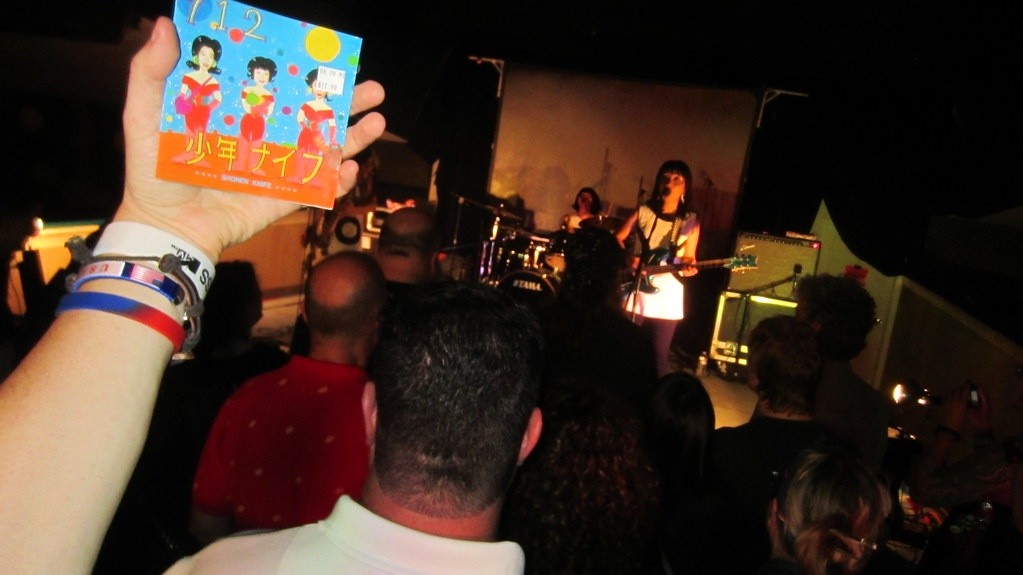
[57,222,216,364]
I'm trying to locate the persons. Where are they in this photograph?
[95,161,1023,575]
[0,16,385,575]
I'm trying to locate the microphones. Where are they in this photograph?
[660,186,671,198]
[793,264,802,288]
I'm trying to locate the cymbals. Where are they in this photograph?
[451,190,522,222]
[578,214,625,231]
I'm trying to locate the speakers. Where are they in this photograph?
[190,260,261,352]
[726,230,821,303]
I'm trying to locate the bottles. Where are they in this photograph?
[694,351,708,377]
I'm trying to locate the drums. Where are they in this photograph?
[484,228,614,299]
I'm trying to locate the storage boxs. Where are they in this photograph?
[710,292,799,368]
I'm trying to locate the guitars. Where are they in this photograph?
[616,246,759,297]
